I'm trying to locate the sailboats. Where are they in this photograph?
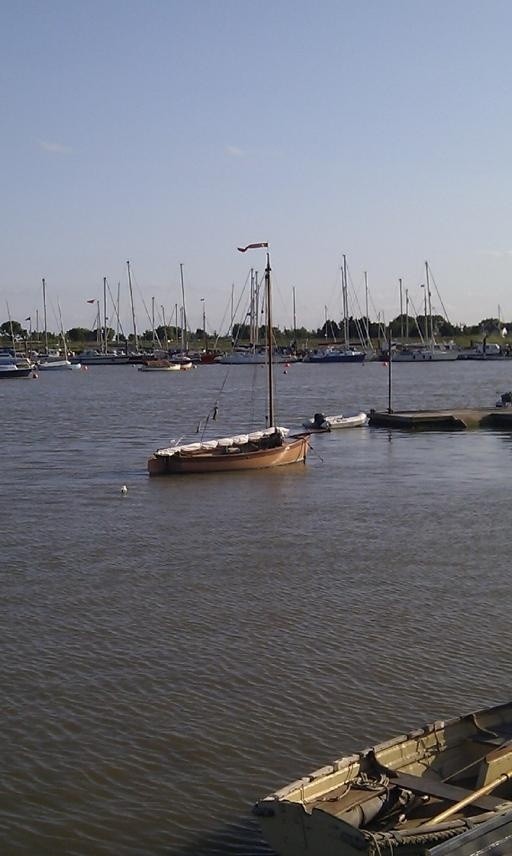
[150,257,310,473]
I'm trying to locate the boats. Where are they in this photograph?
[254,697,510,853]
[303,412,367,427]
